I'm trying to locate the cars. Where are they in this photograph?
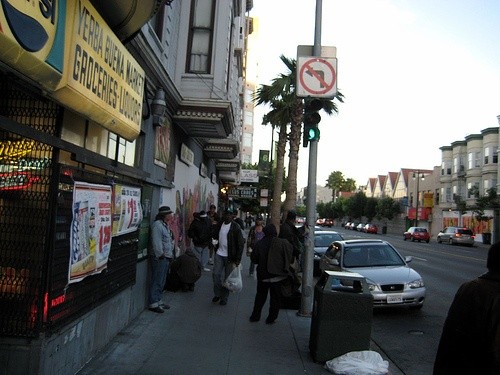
[437,227,475,246]
[302,232,345,273]
[320,239,425,311]
[293,217,379,235]
[404,227,430,244]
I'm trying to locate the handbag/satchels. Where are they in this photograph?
[223,265,243,294]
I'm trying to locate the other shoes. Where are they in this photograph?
[148,304,171,313]
[212,296,228,305]
[203,267,211,272]
[208,258,214,265]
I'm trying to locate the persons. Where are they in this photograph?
[210,210,245,305]
[188,204,305,324]
[432,243,500,375]
[150,206,174,313]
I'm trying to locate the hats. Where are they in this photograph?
[287,209,298,216]
[159,206,172,214]
[224,207,233,214]
[199,210,208,217]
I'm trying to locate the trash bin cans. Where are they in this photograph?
[382,226,387,234]
[308,270,374,364]
[482,233,491,244]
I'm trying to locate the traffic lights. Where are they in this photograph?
[303,97,322,141]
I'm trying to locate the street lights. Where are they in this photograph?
[413,171,425,227]
